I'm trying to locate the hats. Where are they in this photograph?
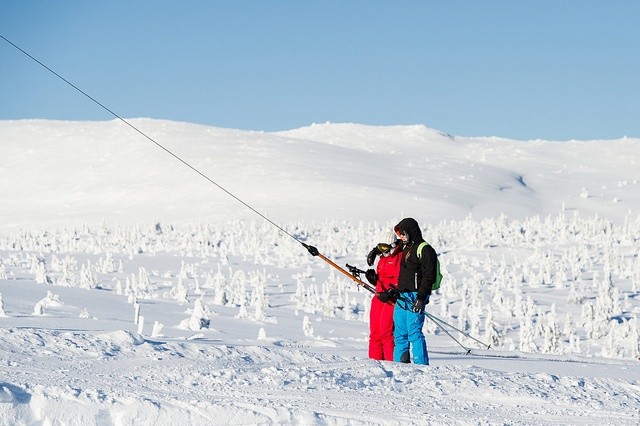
[377,227,397,244]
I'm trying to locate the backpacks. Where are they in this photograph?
[417,242,443,290]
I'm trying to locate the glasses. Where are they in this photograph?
[376,244,390,252]
[399,231,406,236]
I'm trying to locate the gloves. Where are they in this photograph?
[412,297,425,315]
[376,288,396,303]
[365,269,378,286]
[367,248,382,266]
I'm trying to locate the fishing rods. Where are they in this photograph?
[0,33,396,307]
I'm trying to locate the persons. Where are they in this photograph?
[365,231,405,362]
[392,216,437,365]
